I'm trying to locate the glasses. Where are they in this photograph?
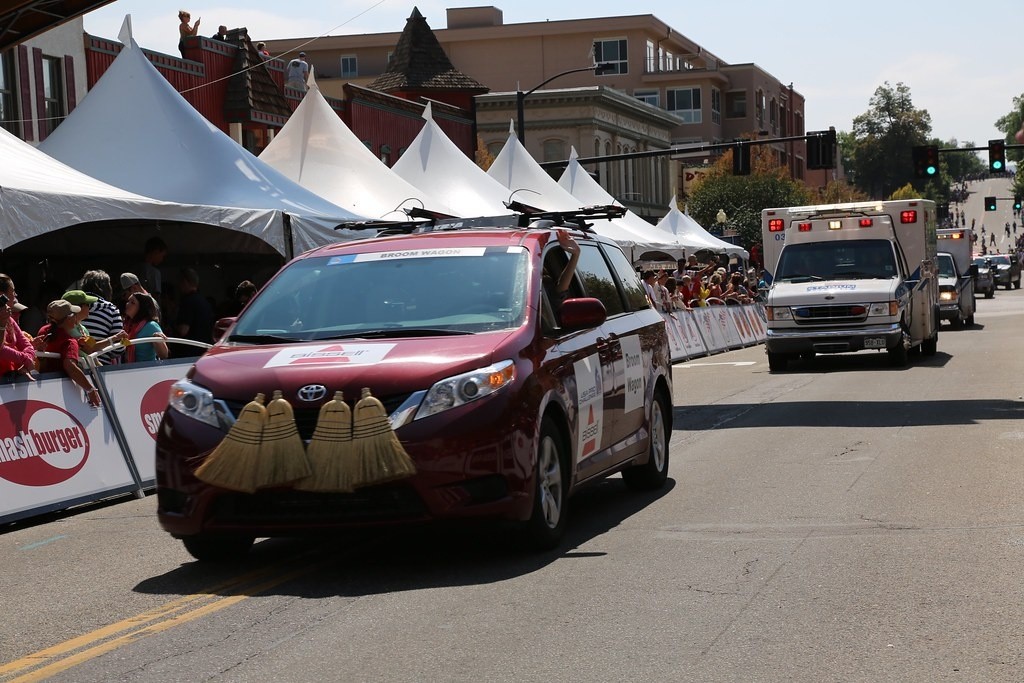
[184,15,190,18]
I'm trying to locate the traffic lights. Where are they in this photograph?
[988,139,1005,173]
[1014,196,1021,209]
[989,197,996,211]
[923,145,940,180]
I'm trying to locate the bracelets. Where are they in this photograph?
[107,337,113,345]
[86,387,98,401]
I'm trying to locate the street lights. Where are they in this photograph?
[517,62,616,147]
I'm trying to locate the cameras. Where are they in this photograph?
[0,294,9,307]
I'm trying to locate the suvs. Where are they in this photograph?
[985,254,1022,290]
[154,204,677,566]
[971,256,998,299]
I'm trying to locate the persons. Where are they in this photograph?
[751,243,763,265]
[178,9,227,59]
[173,265,216,356]
[949,169,1017,235]
[61,290,128,369]
[130,238,166,290]
[973,233,1000,255]
[543,229,581,326]
[218,280,264,333]
[1007,233,1024,270]
[37,300,101,408]
[727,266,766,304]
[121,273,161,326]
[635,254,732,313]
[80,270,126,366]
[125,292,169,362]
[0,273,41,379]
[256,42,309,90]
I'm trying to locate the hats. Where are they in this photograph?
[13,303,28,312]
[47,300,81,320]
[61,289,98,304]
[676,280,685,286]
[300,52,306,56]
[120,272,139,290]
[643,270,657,278]
[145,237,167,253]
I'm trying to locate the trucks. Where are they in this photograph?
[761,198,942,375]
[937,226,980,330]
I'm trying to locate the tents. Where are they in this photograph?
[31,14,366,219]
[0,127,375,263]
[258,65,467,221]
[389,101,529,218]
[485,118,745,274]
